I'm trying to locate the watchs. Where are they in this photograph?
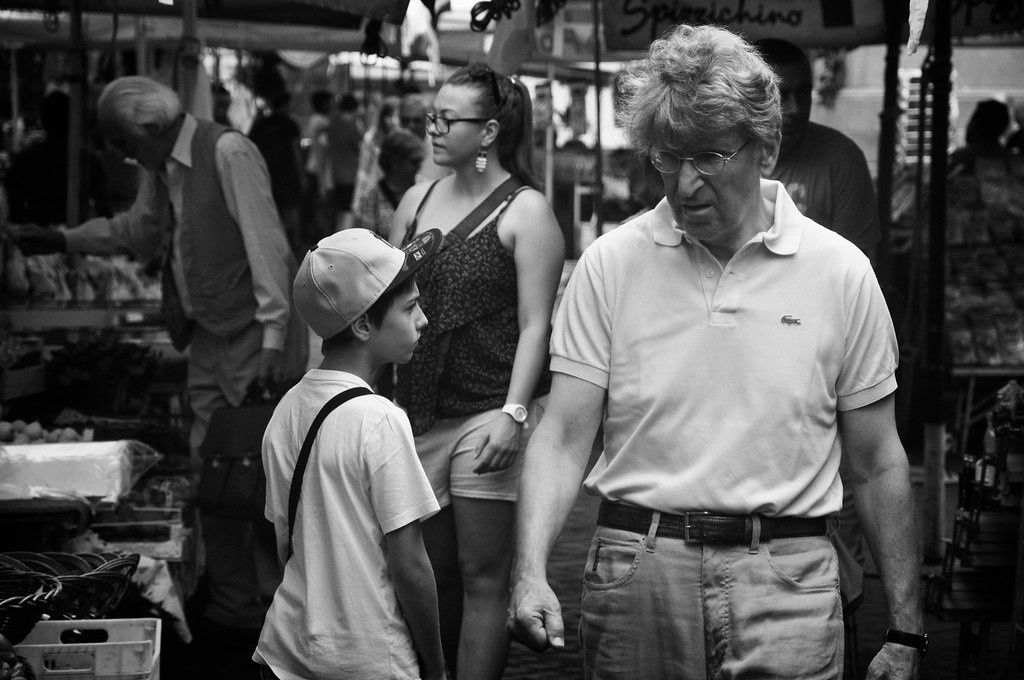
[502,404,528,423]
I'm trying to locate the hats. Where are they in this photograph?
[291,226,444,342]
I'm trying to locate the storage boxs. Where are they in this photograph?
[13,617,161,680]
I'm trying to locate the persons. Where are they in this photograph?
[4,77,308,621]
[244,91,429,236]
[5,90,112,227]
[210,82,232,126]
[503,24,927,680]
[951,99,1024,178]
[755,37,881,274]
[251,227,446,680]
[389,64,565,680]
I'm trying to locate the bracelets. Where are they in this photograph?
[883,629,928,654]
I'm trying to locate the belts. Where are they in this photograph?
[594,499,829,548]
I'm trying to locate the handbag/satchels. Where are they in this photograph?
[197,376,284,524]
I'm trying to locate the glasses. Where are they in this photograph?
[645,139,754,177]
[423,113,490,135]
